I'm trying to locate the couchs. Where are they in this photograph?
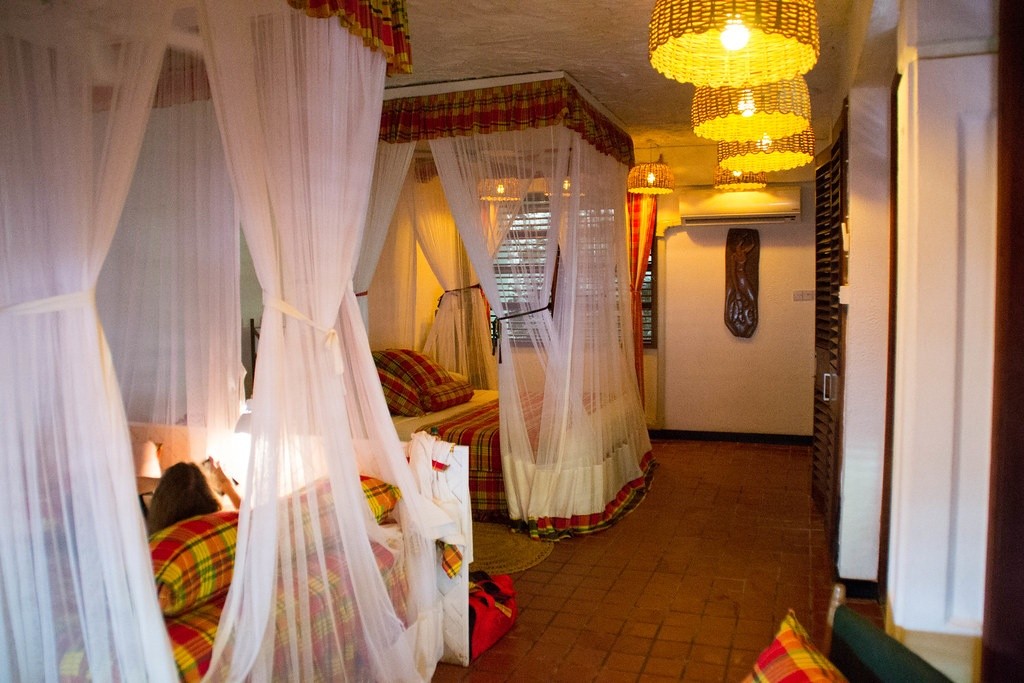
[59,469,408,683]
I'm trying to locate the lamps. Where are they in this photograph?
[717,126,816,175]
[691,72,812,142]
[648,0,821,88]
[627,139,674,195]
[137,440,162,479]
[543,176,586,197]
[712,165,767,192]
[477,176,521,201]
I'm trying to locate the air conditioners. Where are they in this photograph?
[678,184,801,227]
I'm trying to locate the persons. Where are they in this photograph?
[142,458,243,532]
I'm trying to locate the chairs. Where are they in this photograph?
[822,584,957,683]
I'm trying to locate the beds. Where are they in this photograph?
[249,67,661,543]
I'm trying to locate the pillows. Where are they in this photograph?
[377,368,426,418]
[150,511,239,616]
[371,348,455,393]
[421,381,475,412]
[742,608,849,683]
[276,473,402,577]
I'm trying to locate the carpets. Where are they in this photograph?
[469,522,555,575]
